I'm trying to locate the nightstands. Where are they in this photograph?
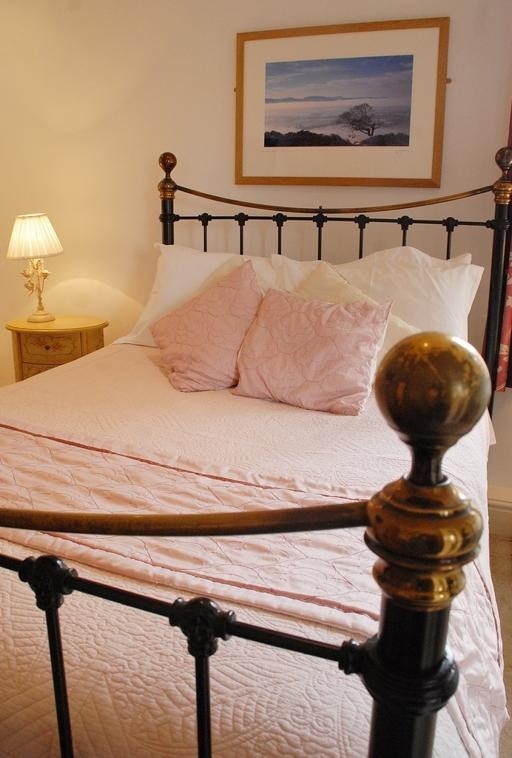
[6,308,109,389]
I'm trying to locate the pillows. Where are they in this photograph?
[271,246,485,387]
[113,241,269,352]
[237,276,392,420]
[152,254,262,394]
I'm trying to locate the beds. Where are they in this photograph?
[2,141,512,756]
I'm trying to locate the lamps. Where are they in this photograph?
[5,205,66,325]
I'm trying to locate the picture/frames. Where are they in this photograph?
[233,16,451,188]
